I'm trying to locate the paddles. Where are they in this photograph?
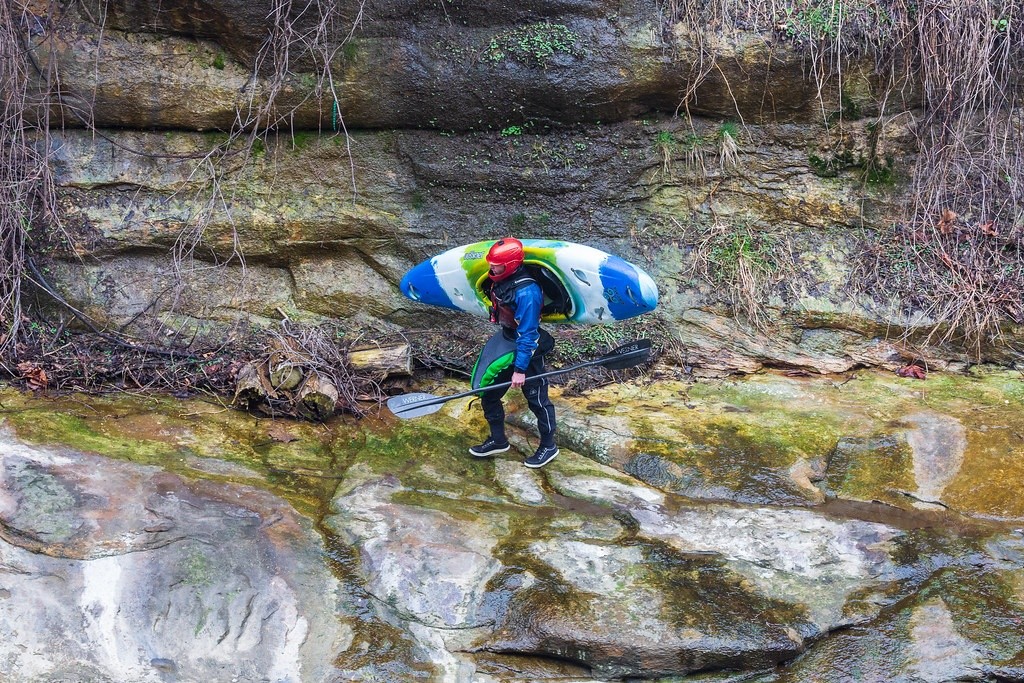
[386,338,653,422]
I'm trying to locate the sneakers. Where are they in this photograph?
[469,435,511,457]
[524,443,559,469]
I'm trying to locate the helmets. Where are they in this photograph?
[486,237,525,282]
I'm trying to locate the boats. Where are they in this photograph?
[398,239,659,324]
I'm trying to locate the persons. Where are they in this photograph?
[466,238,561,469]
